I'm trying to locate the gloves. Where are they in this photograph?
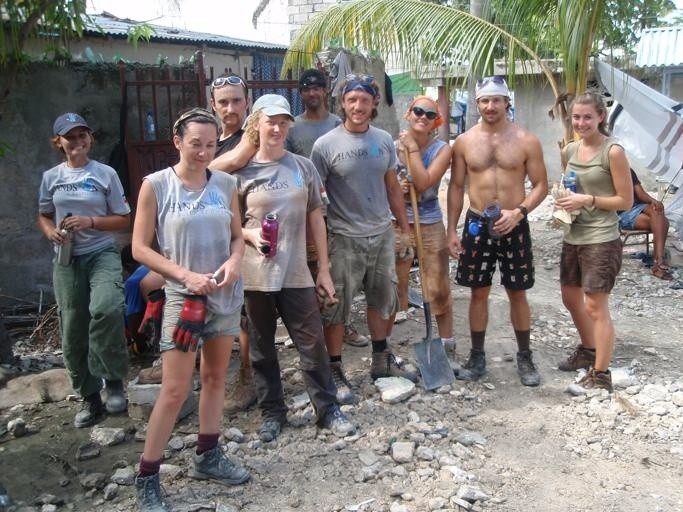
[173,295,206,353]
[137,288,167,347]
[551,184,580,224]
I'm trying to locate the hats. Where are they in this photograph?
[300,68,327,90]
[253,94,296,121]
[475,76,511,100]
[53,112,94,135]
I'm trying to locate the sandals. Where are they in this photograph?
[654,256,668,272]
[650,265,673,279]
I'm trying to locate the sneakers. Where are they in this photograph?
[187,445,250,485]
[138,363,164,384]
[516,350,541,386]
[133,470,167,512]
[75,392,101,427]
[329,361,354,405]
[370,347,417,383]
[567,368,614,395]
[343,330,369,347]
[322,407,356,436]
[443,340,461,376]
[558,345,597,371]
[223,384,258,414]
[459,349,487,381]
[259,417,289,442]
[104,378,126,412]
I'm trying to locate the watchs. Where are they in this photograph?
[516,205,528,219]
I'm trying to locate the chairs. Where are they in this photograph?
[614,209,654,260]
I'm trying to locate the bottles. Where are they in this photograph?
[260,215,280,258]
[484,201,504,242]
[144,111,156,142]
[561,172,576,194]
[56,212,75,268]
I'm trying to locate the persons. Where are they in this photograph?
[552,90,633,397]
[616,167,673,280]
[132,108,250,512]
[447,75,548,386]
[206,68,457,441]
[38,112,131,428]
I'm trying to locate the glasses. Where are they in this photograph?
[410,106,437,120]
[478,76,504,87]
[211,77,245,88]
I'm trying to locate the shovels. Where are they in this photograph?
[398,138,456,392]
[407,286,424,310]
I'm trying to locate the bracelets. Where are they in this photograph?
[592,195,595,207]
[88,216,94,229]
